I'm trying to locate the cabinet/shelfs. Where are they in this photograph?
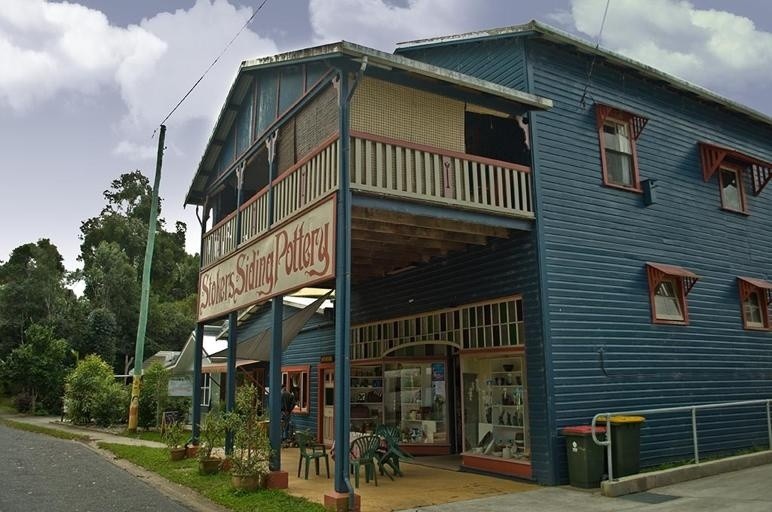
[487,356,529,457]
[348,364,423,443]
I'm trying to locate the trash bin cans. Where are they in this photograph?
[557,415,645,490]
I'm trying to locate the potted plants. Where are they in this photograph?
[231,427,270,493]
[160,421,186,461]
[197,411,230,475]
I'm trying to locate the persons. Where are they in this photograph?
[281,384,296,442]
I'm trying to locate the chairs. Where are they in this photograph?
[294,432,330,480]
[349,424,418,488]
